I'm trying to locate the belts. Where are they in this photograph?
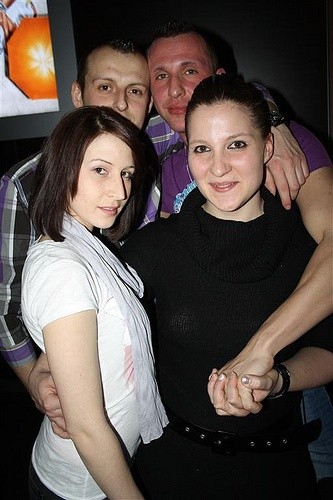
[165,412,322,455]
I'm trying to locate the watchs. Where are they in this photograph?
[269,111,284,126]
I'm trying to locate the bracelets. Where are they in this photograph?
[268,363,290,399]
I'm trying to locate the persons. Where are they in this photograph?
[148,22,333,416]
[22,107,170,500]
[0,41,309,389]
[29,75,333,500]
